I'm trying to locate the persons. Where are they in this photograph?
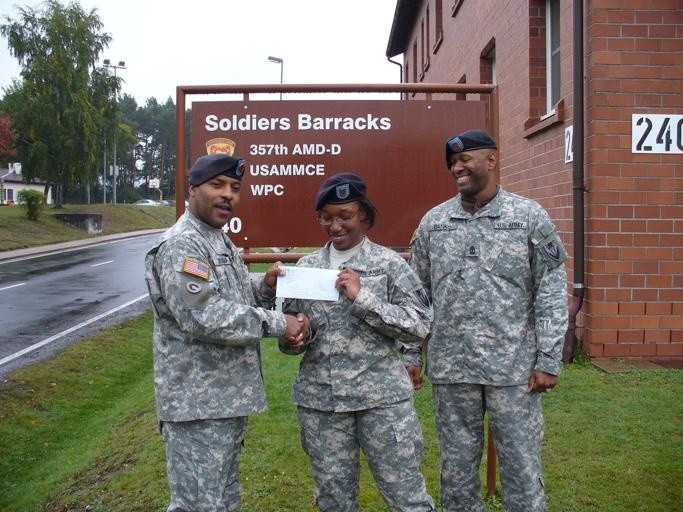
[273,170,438,512]
[402,128,568,512]
[143,151,313,511]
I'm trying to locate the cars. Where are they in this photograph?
[132,199,189,207]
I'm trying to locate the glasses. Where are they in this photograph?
[318,207,360,225]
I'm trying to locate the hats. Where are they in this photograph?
[445,129,497,171]
[186,153,245,187]
[314,172,365,211]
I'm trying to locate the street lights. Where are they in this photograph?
[267,56,284,100]
[101,59,127,205]
